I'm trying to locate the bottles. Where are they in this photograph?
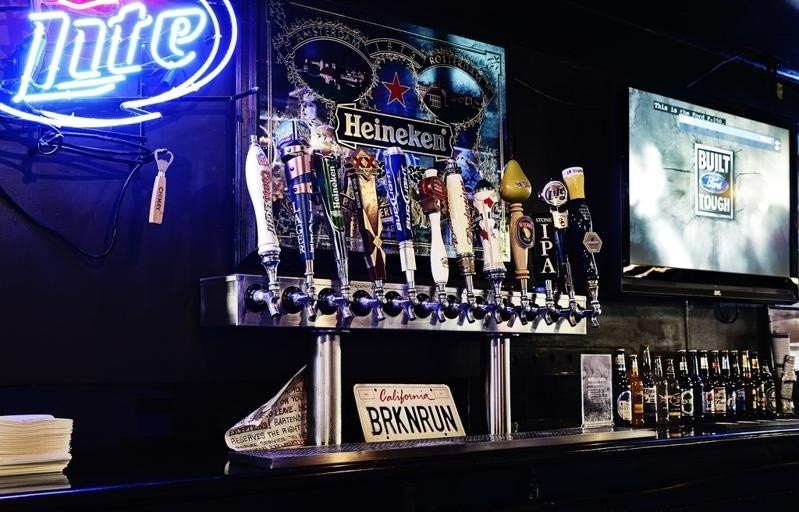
[612,343,778,427]
